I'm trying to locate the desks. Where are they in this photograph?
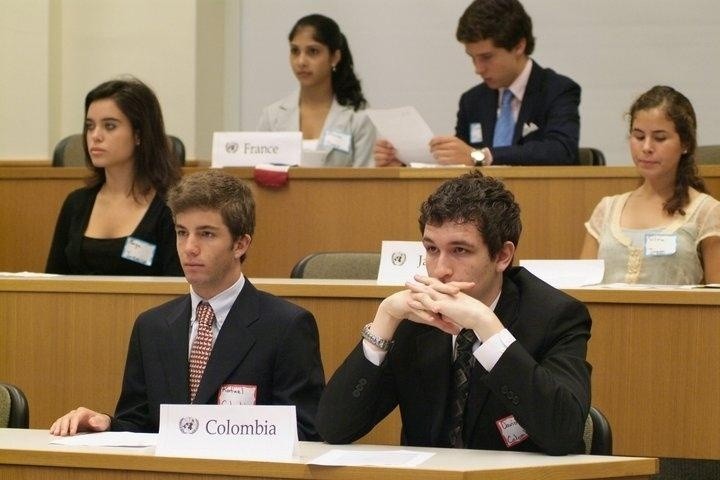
[1,425,662,480]
[0,157,720,285]
[0,275,720,465]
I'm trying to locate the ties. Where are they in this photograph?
[189,303,215,402]
[492,90,515,147]
[447,329,478,448]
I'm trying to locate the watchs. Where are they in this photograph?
[361,321,397,353]
[469,148,488,168]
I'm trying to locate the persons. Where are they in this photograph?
[49,168,325,441]
[43,73,188,276]
[373,0,584,168]
[574,85,720,287]
[252,13,380,168]
[312,167,593,457]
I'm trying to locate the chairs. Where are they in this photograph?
[50,132,187,168]
[577,146,607,165]
[0,382,31,428]
[286,248,380,280]
[583,407,614,456]
[692,143,720,166]
